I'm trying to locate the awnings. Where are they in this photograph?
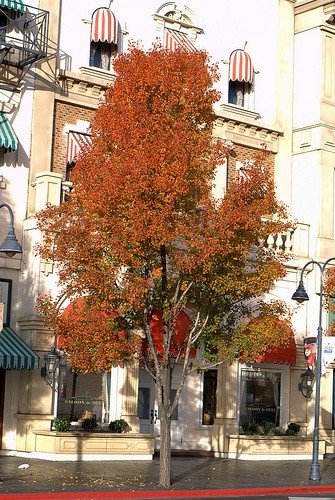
[68,130,94,167]
[238,316,296,369]
[54,294,137,355]
[238,168,269,192]
[0,327,40,372]
[91,8,118,45]
[0,0,26,13]
[0,112,19,151]
[164,27,200,55]
[229,50,254,86]
[140,305,197,359]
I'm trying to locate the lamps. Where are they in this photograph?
[297,364,316,398]
[40,345,63,390]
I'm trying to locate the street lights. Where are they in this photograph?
[292,258,335,481]
